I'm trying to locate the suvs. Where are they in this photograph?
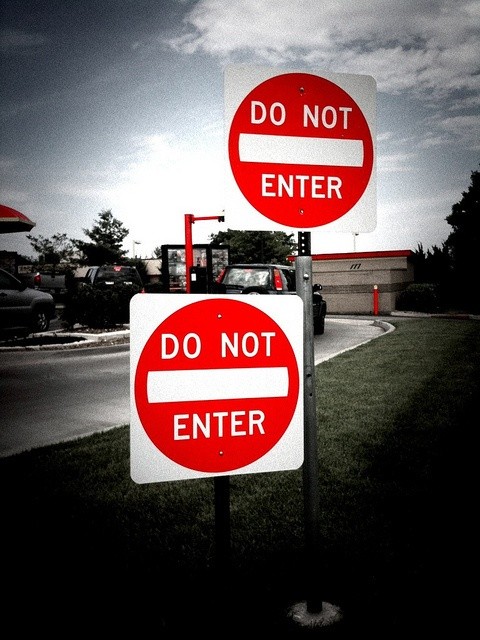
[215,262,328,341]
[0,268,56,337]
[80,264,145,293]
[34,270,65,290]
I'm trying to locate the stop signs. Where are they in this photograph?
[128,291,308,486]
[224,64,381,236]
